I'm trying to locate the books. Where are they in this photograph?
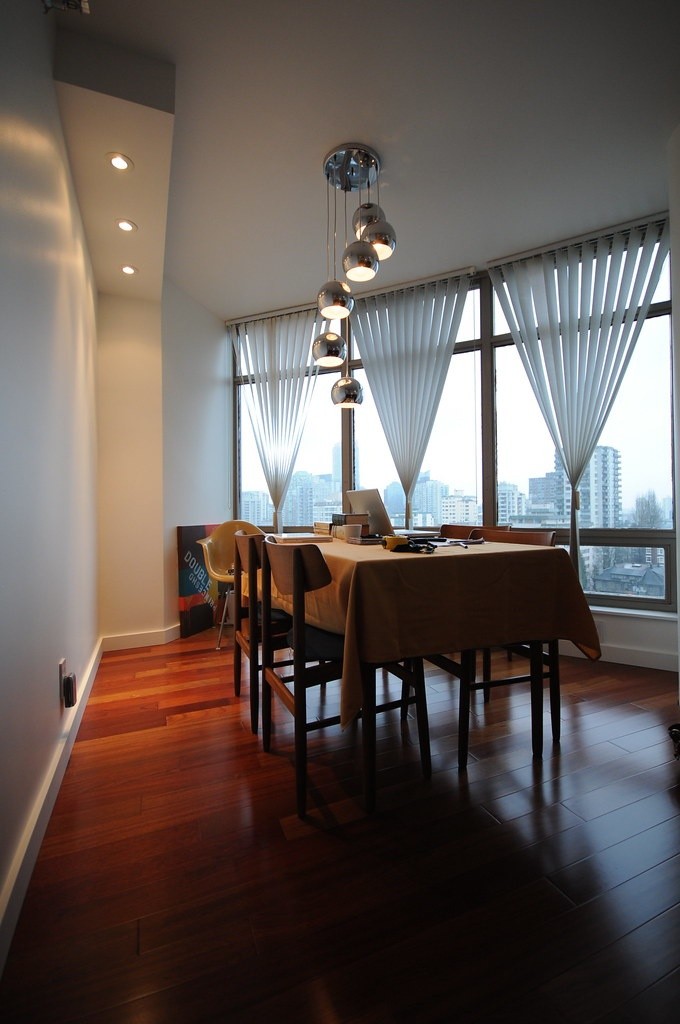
[273,513,383,545]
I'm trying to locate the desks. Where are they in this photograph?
[241,532,602,814]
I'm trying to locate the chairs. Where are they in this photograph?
[262,540,432,819]
[196,520,266,649]
[401,526,561,767]
[233,528,325,697]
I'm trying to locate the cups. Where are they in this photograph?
[343,525,362,538]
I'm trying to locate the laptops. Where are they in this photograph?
[347,488,441,538]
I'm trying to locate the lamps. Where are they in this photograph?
[311,142,395,408]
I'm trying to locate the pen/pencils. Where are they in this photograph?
[460,542,468,549]
[408,539,447,542]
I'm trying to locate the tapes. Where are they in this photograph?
[382,535,408,549]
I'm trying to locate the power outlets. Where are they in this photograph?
[58,658,66,700]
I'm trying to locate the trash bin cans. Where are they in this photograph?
[225,590,235,621]
